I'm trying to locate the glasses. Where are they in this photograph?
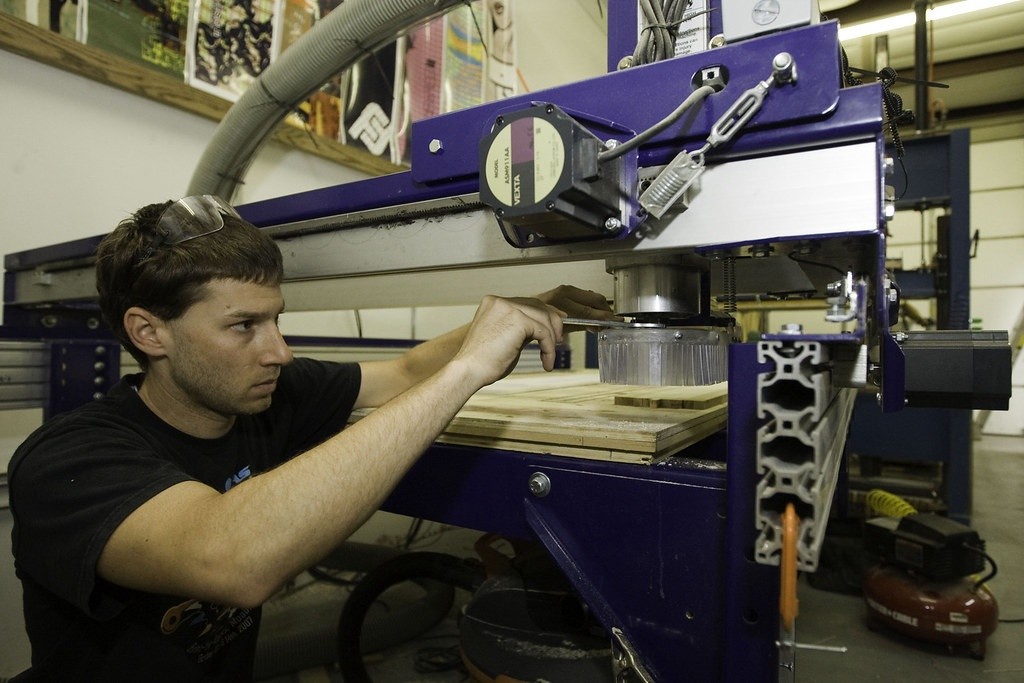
[136,194,262,270]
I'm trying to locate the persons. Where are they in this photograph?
[3,195,629,683]
[888,298,933,333]
